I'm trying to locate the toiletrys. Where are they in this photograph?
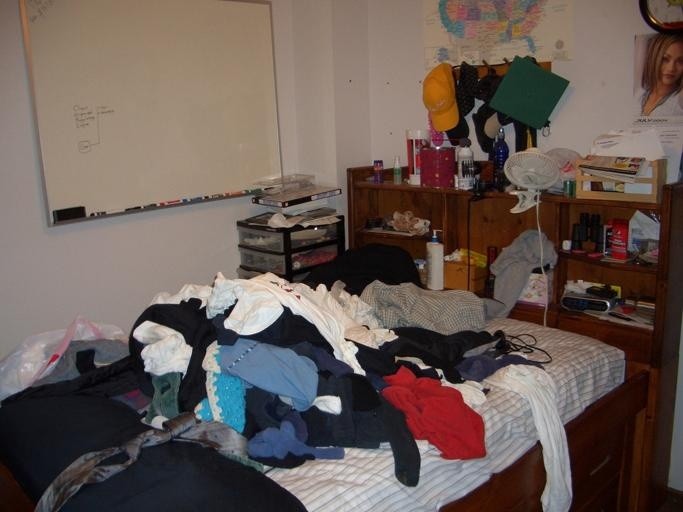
[393,156,402,185]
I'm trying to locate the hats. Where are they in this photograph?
[423,62,460,132]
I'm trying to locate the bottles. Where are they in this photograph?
[484,246,498,298]
[393,128,480,191]
[492,127,510,192]
[571,212,609,253]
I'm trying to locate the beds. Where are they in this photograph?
[1,303,651,512]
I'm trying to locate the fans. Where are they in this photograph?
[505,148,559,214]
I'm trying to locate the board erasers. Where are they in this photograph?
[53,206,86,224]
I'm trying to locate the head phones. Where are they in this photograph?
[494,330,511,355]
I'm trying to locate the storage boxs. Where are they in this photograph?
[443,260,488,294]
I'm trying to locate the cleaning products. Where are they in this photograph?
[457,148,474,189]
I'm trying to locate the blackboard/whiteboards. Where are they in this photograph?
[18,0,285,229]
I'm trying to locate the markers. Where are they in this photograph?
[90,187,275,216]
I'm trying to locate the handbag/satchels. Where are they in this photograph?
[489,56,570,131]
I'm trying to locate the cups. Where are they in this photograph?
[562,240,572,250]
[562,178,576,200]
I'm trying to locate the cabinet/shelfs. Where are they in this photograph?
[236,211,345,288]
[346,165,683,497]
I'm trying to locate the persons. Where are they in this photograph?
[634,32,683,117]
